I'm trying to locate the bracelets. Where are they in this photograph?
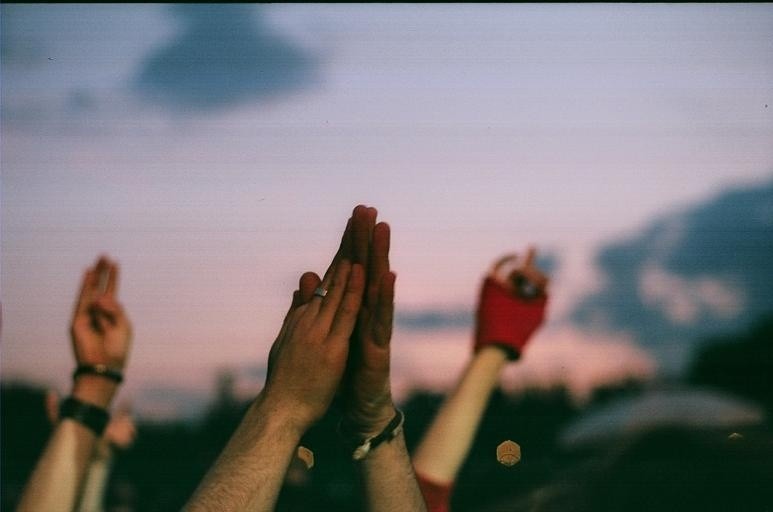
[336,406,405,460]
[57,396,110,438]
[72,362,122,387]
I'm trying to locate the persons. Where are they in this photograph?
[410,246,550,511]
[175,204,428,511]
[13,256,133,512]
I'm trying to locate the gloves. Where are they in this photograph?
[475,276,548,361]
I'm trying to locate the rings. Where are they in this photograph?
[312,286,327,297]
